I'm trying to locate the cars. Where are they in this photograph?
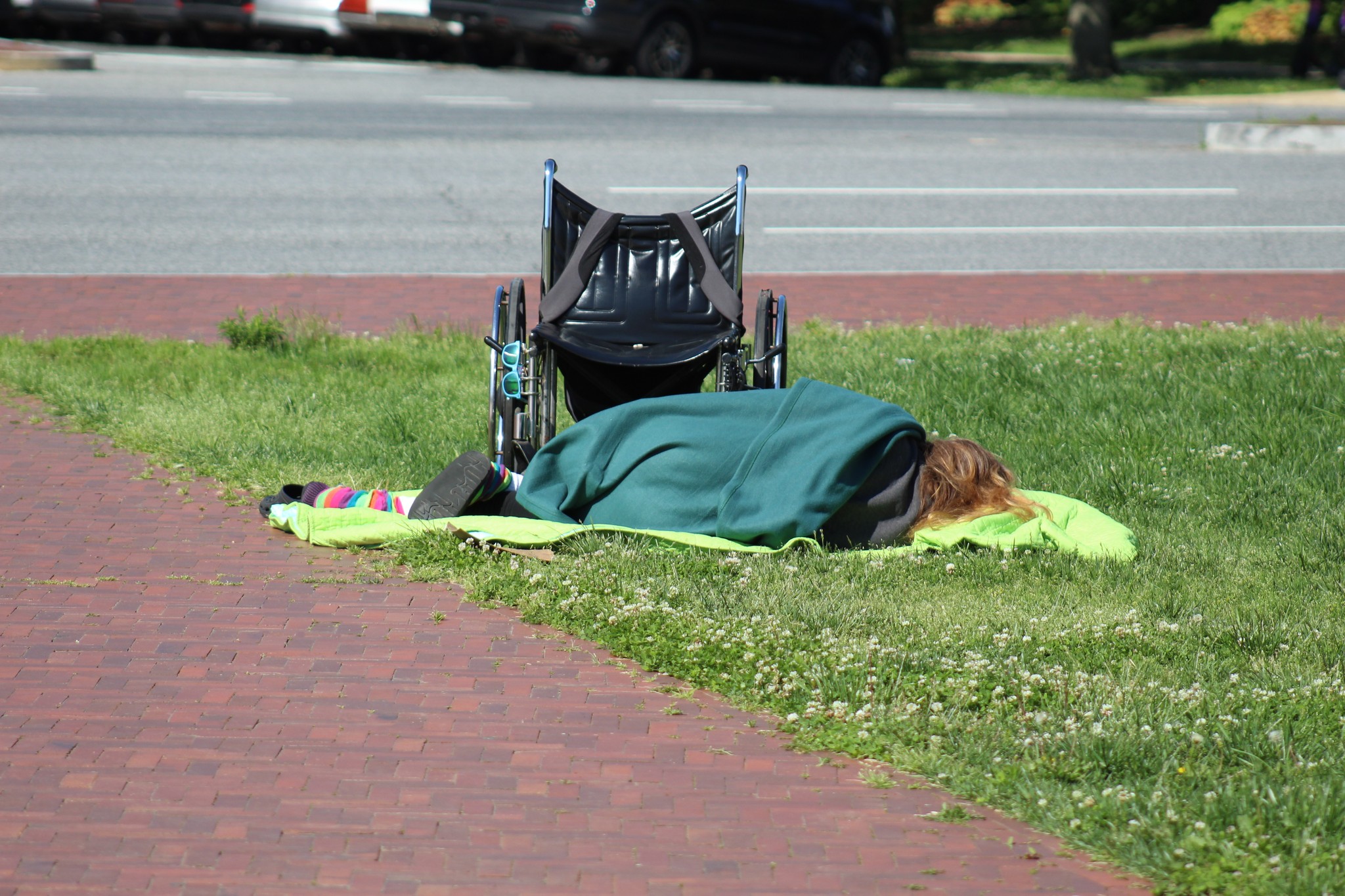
[0,0,916,92]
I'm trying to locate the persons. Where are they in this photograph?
[409,379,1052,552]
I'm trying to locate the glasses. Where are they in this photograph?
[502,341,521,399]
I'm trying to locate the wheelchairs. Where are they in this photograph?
[482,158,789,465]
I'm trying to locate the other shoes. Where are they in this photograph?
[408,450,493,520]
